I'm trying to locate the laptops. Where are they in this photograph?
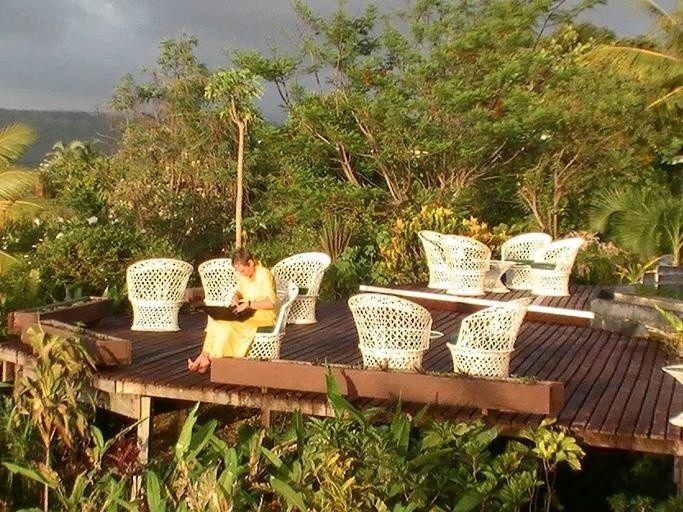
[201,304,256,322]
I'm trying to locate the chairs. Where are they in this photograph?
[270,252,331,324]
[126,257,194,332]
[417,230,585,298]
[446,297,531,378]
[227,279,299,360]
[197,258,240,332]
[348,294,444,369]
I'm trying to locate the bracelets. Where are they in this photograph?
[248,300,250,308]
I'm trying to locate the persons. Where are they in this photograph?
[185,246,279,376]
